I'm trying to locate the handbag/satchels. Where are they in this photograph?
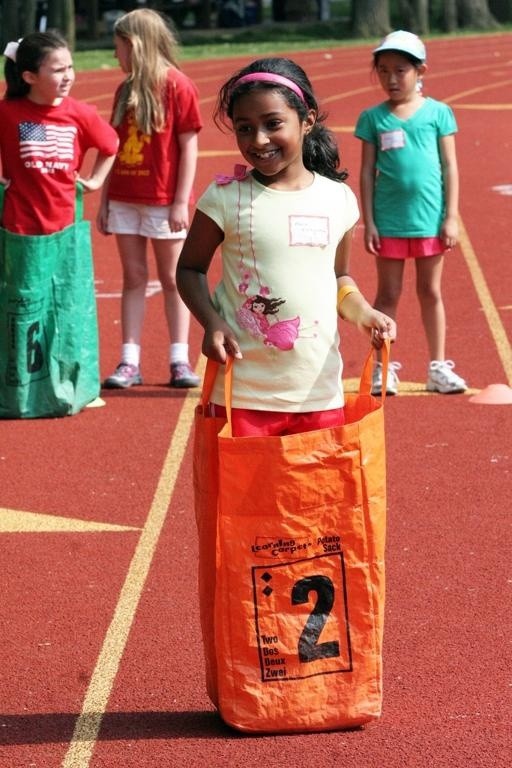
[194,390,388,734]
[0,219,101,419]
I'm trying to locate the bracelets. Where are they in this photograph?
[338,284,360,321]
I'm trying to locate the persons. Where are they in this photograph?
[175,58,398,440]
[354,29,470,397]
[96,7,204,389]
[1,30,121,236]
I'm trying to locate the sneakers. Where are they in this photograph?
[104,362,142,388]
[169,361,200,387]
[372,30,427,65]
[369,358,402,393]
[426,360,468,393]
